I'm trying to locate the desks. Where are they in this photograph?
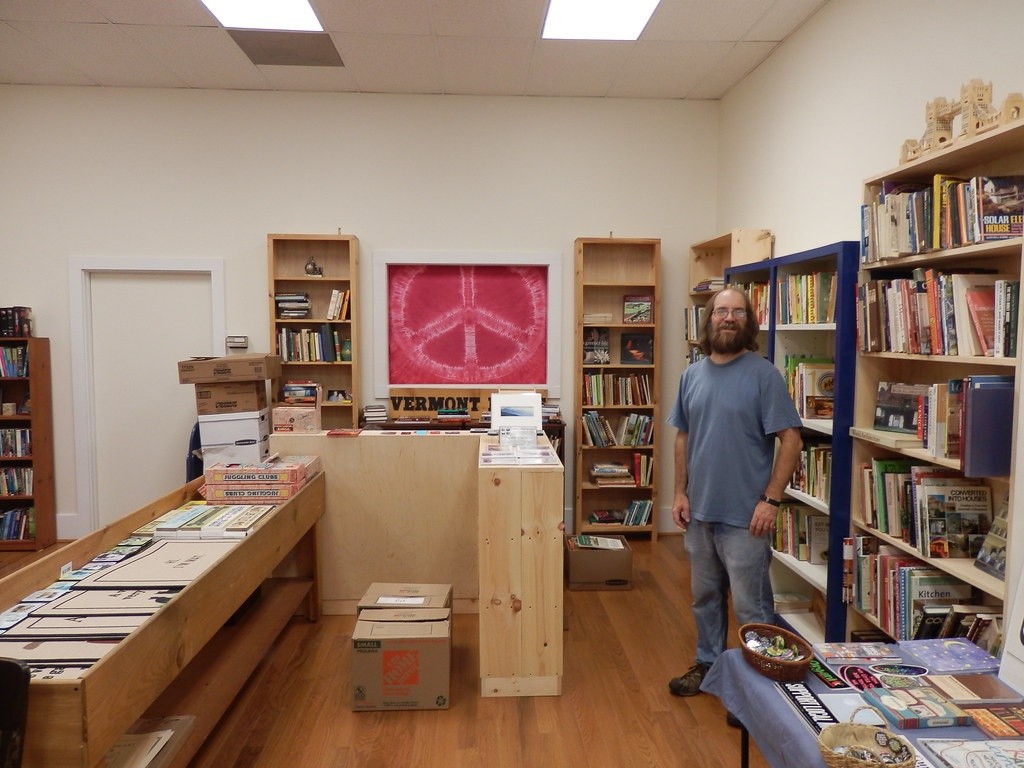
[699,637,1024,768]
[0,457,326,768]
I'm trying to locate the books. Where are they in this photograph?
[858,172,1024,358]
[843,534,1002,654]
[577,534,624,550]
[275,290,312,319]
[362,405,388,423]
[283,379,316,404]
[0,306,34,541]
[872,373,1014,479]
[278,327,323,362]
[583,296,654,448]
[320,324,341,362]
[774,637,1024,768]
[393,408,472,424]
[327,428,363,437]
[860,457,1009,583]
[588,452,653,526]
[542,404,560,422]
[773,353,835,565]
[326,290,350,320]
[728,272,837,324]
[683,276,724,367]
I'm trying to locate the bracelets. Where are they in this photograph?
[762,495,781,508]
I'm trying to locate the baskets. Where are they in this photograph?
[738,622,812,681]
[818,705,916,768]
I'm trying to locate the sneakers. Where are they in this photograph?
[669,660,713,696]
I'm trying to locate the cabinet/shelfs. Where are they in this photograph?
[847,115,1024,678]
[574,236,663,542]
[724,240,860,646]
[684,232,772,554]
[267,232,361,432]
[0,336,57,551]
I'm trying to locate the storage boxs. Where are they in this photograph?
[351,581,453,711]
[178,352,282,477]
[564,535,632,590]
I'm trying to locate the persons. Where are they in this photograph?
[328,391,346,400]
[665,287,804,728]
